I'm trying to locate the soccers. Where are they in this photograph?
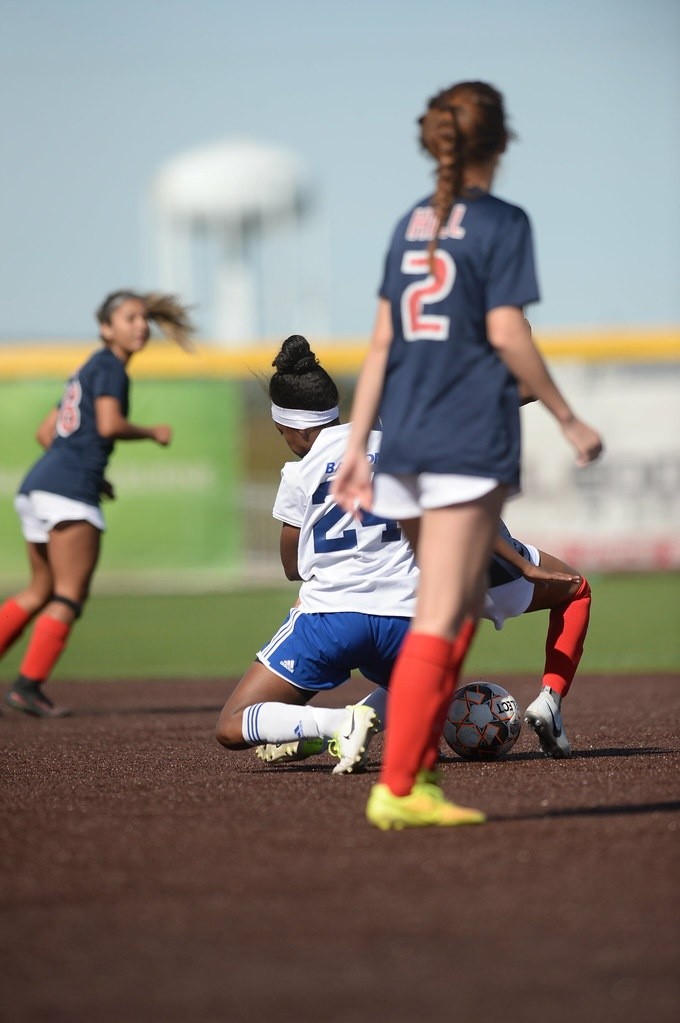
[442,680,521,760]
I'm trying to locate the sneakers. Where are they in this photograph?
[253,738,309,764]
[327,705,382,775]
[3,686,72,718]
[522,686,571,760]
[364,783,486,831]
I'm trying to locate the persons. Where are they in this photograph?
[0,292,198,717]
[329,77,605,828]
[214,334,419,773]
[482,322,591,755]
